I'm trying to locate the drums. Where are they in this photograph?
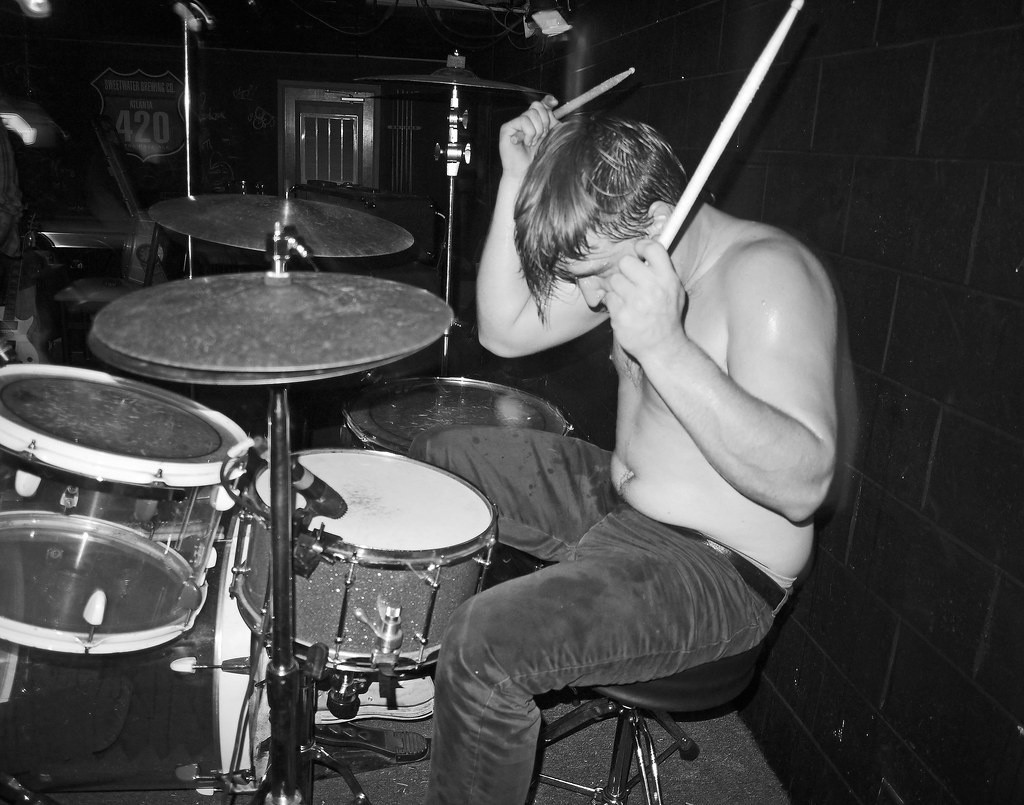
[0,509,209,792]
[339,376,574,458]
[0,363,256,541]
[228,448,497,681]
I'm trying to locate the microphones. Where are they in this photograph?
[254,436,349,521]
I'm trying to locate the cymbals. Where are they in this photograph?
[147,193,415,258]
[93,271,455,373]
[85,326,434,386]
[352,67,554,99]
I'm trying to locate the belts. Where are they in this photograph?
[682,528,791,617]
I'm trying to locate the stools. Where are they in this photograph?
[534,645,761,805]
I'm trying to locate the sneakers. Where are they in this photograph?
[315,676,434,724]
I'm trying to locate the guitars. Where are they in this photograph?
[0,208,39,368]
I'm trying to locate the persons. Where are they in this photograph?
[409,97,839,805]
